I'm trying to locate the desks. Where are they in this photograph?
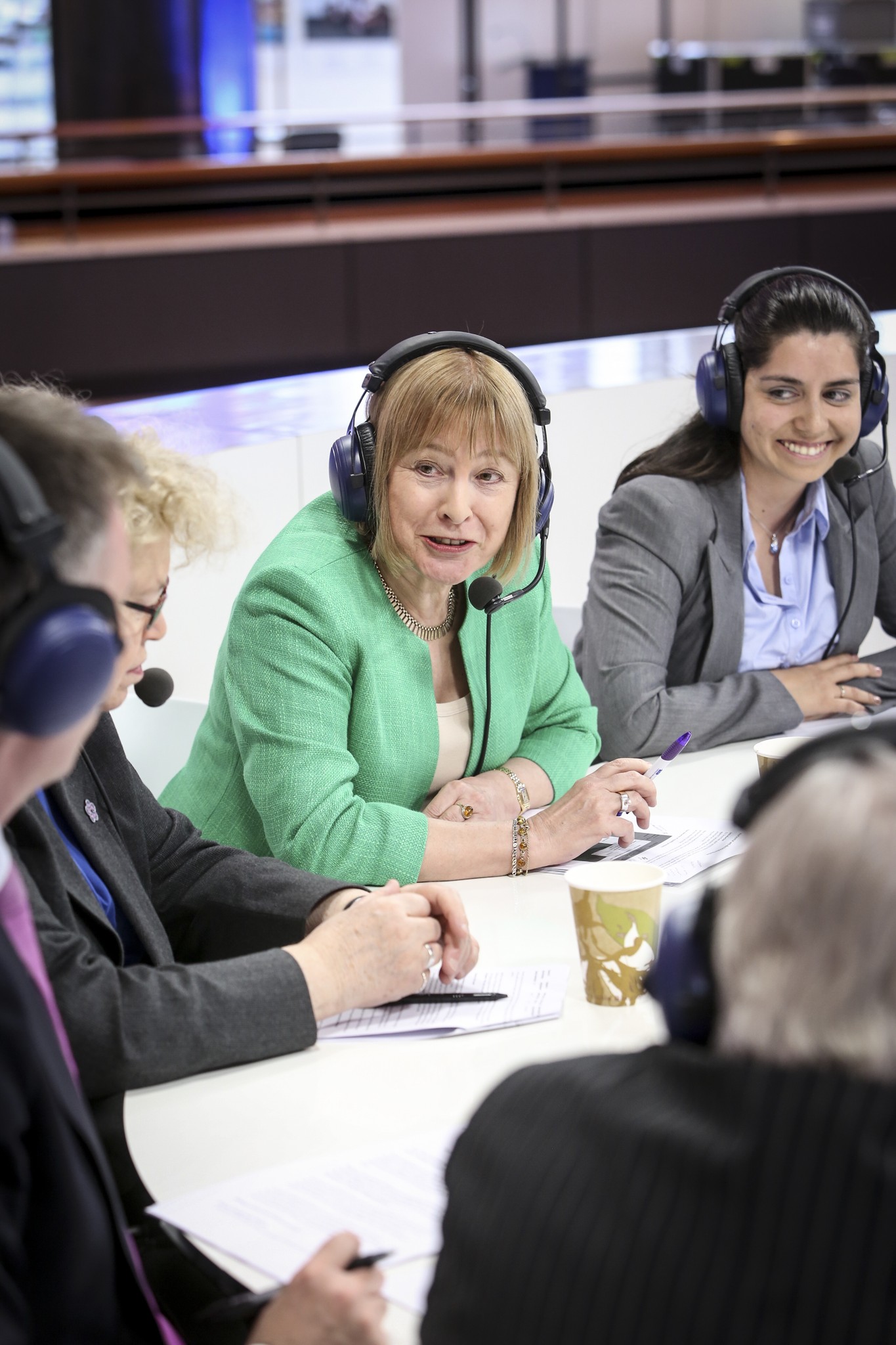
[120,714,849,1345]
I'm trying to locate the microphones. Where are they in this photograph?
[467,536,547,614]
[832,425,888,487]
[134,668,174,708]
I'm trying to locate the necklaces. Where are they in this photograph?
[748,510,791,554]
[369,548,459,641]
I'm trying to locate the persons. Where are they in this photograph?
[0,387,480,1345]
[153,332,657,881]
[569,267,896,761]
[421,739,896,1345]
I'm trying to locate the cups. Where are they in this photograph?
[564,859,665,1009]
[754,736,815,778]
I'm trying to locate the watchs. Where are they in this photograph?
[493,767,530,816]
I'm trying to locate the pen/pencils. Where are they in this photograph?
[642,731,692,783]
[377,990,509,1005]
[202,1254,389,1318]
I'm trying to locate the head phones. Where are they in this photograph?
[694,266,889,458]
[0,437,125,737]
[642,717,896,1047]
[328,331,554,543]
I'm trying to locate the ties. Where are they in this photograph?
[36,787,117,930]
[0,862,183,1344]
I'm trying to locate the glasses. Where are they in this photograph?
[120,577,171,634]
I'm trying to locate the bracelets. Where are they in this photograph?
[508,816,531,876]
[343,895,366,910]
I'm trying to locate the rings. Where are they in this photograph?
[425,944,434,970]
[615,791,632,812]
[839,684,845,698]
[416,973,427,994]
[454,803,473,819]
